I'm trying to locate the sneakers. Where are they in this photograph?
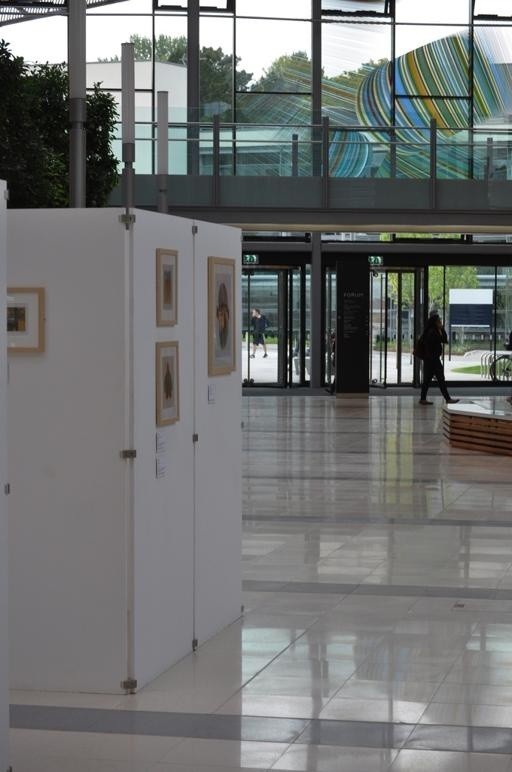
[419,399,434,405]
[446,397,460,405]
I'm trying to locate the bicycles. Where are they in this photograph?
[489,342,512,387]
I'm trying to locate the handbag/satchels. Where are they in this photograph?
[413,333,426,361]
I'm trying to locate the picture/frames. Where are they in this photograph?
[155,340,180,428]
[6,286,44,352]
[156,248,178,328]
[206,255,238,376]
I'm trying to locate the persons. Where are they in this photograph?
[418,314,460,405]
[248,307,272,359]
